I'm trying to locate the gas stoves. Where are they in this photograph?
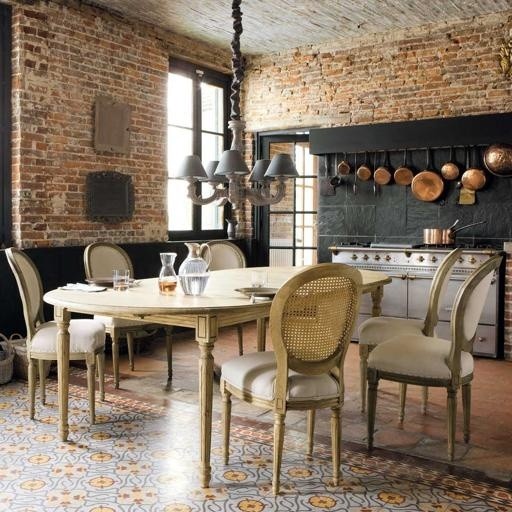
[329,240,497,269]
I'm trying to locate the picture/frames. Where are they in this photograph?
[86,170,135,222]
[94,95,130,154]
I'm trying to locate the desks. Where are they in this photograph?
[42,265,392,487]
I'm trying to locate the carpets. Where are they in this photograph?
[0,364,512,512]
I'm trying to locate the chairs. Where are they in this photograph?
[4,247,104,425]
[83,242,173,390]
[357,248,463,421]
[366,254,503,462]
[201,239,247,356]
[218,262,363,497]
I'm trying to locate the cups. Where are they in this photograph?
[247,268,268,291]
[112,270,129,291]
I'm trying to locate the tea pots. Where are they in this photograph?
[181,241,212,296]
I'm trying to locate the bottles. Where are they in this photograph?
[155,252,177,294]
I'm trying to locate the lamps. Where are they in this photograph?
[179,1,299,207]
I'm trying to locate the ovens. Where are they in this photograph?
[329,269,499,326]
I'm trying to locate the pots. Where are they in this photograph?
[410,148,444,201]
[423,216,486,248]
[394,149,412,186]
[442,146,460,182]
[358,153,370,181]
[461,147,486,190]
[376,155,391,188]
[483,144,511,177]
[338,155,351,175]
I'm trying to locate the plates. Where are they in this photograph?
[235,288,289,301]
[86,278,133,286]
